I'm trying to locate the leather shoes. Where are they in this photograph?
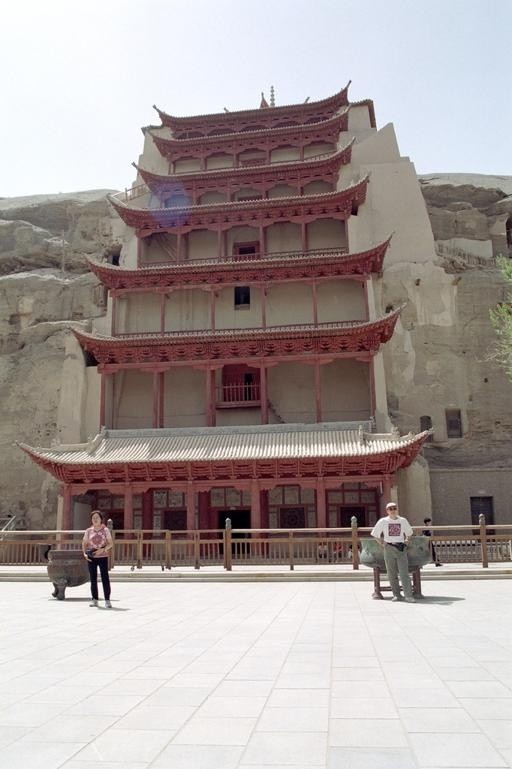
[436,563,442,567]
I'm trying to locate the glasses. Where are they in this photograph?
[387,508,397,510]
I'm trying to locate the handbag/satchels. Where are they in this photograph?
[396,543,406,552]
[86,550,92,557]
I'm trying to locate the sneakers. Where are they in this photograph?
[392,594,416,603]
[89,598,98,607]
[105,600,112,608]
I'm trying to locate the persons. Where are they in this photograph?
[316,541,362,563]
[422,517,442,568]
[82,511,113,608]
[370,503,416,603]
